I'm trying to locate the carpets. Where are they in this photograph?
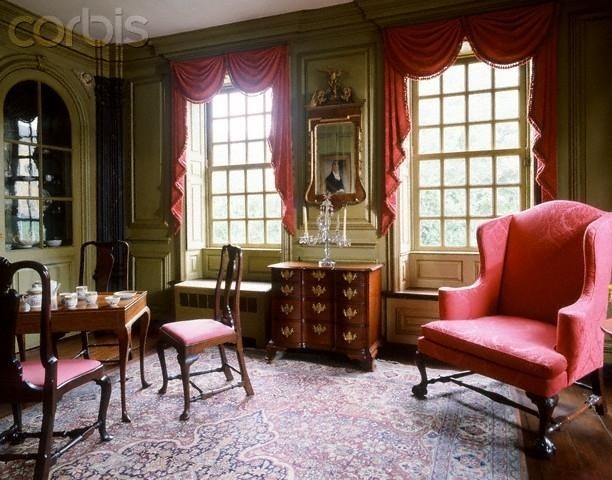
[0,339,529,480]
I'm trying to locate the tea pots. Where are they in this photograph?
[31,279,61,311]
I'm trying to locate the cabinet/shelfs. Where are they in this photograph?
[265,258,385,372]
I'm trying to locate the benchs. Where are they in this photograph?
[172,279,272,348]
[383,287,476,350]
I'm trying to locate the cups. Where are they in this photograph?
[22,302,31,312]
[59,286,98,308]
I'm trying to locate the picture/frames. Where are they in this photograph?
[317,153,352,193]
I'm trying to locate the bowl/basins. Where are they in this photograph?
[104,296,121,307]
[46,239,63,248]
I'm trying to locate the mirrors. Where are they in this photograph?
[303,99,367,213]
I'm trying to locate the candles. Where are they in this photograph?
[302,204,308,238]
[342,204,348,242]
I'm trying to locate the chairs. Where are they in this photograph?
[78,240,133,364]
[0,256,114,479]
[155,244,254,421]
[415,199,612,455]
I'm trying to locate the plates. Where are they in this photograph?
[113,290,138,300]
[16,245,33,249]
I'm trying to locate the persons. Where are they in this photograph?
[325,160,345,193]
